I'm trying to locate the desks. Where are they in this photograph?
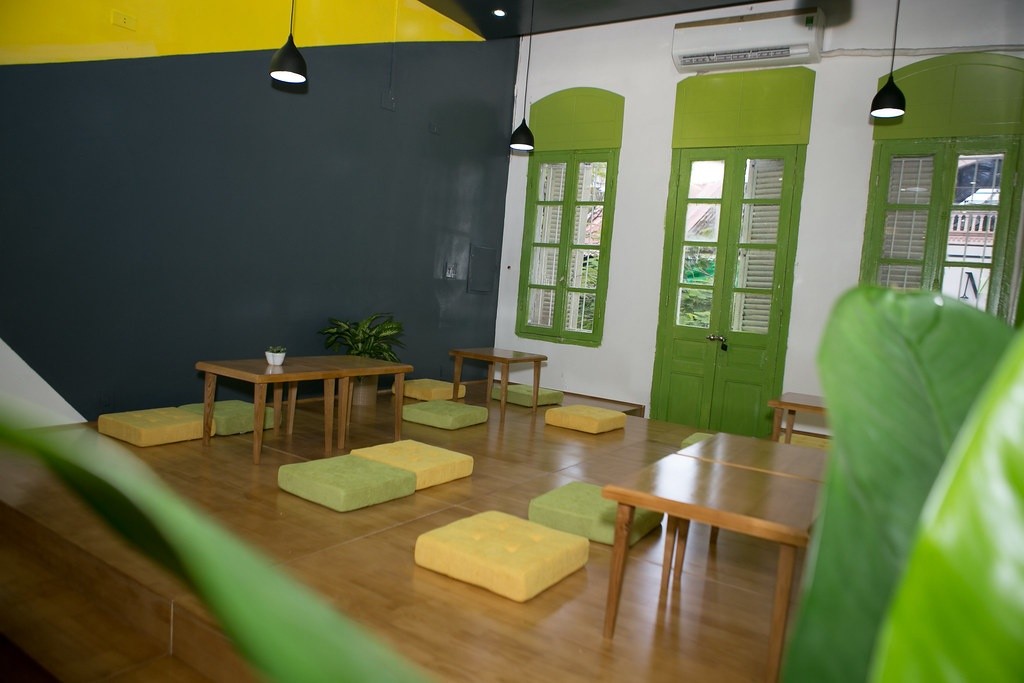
[767,391,828,444]
[600,452,822,683]
[448,348,548,422]
[672,431,829,591]
[195,359,345,465]
[285,355,414,450]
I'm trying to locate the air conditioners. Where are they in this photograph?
[671,6,827,74]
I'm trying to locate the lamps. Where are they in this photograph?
[492,0,511,18]
[509,0,534,152]
[870,0,906,118]
[268,0,307,84]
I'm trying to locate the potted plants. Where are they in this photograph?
[265,344,287,366]
[316,311,408,405]
